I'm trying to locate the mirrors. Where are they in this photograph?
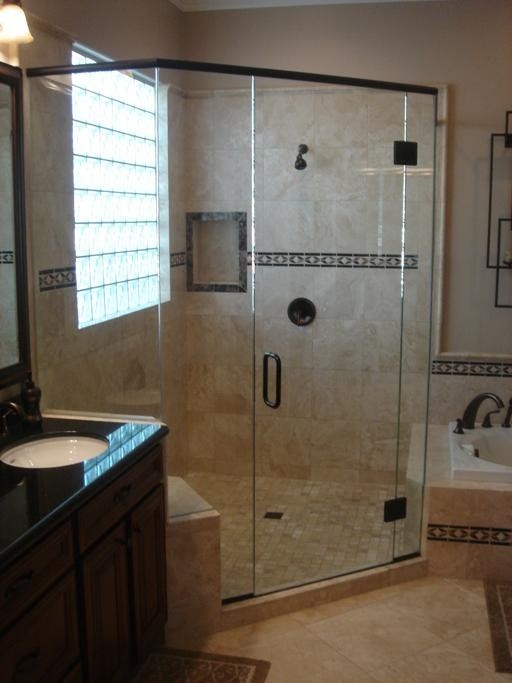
[0,60,33,391]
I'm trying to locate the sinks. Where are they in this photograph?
[0,433,111,469]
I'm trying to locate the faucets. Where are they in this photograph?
[461,393,503,430]
[0,398,25,417]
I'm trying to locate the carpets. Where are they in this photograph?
[483,579,512,672]
[129,646,271,683]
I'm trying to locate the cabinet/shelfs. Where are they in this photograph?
[81,444,167,681]
[2,520,85,680]
